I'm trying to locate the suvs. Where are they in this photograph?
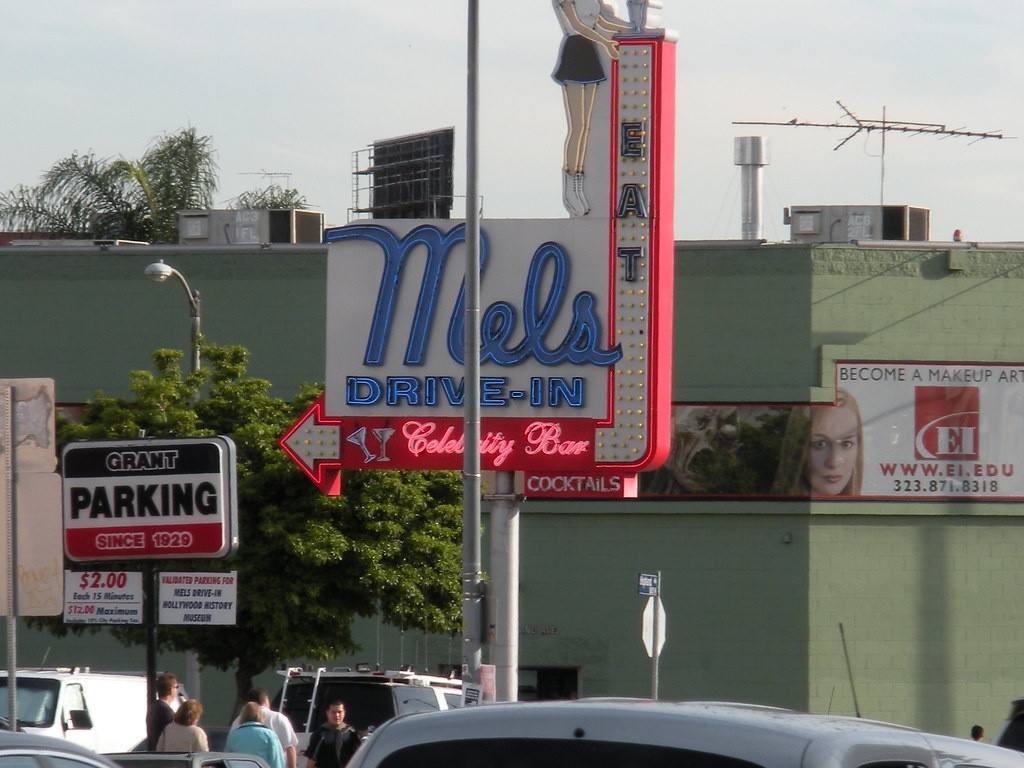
[266,668,467,768]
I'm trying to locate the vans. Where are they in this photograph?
[1,667,184,758]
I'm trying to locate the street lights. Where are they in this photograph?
[145,259,204,412]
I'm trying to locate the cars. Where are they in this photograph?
[0,730,269,768]
[332,697,1024,768]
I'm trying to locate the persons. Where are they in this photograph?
[772,387,864,496]
[970,725,985,741]
[303,700,375,768]
[223,702,285,768]
[146,674,177,751]
[231,688,299,768]
[156,699,212,768]
